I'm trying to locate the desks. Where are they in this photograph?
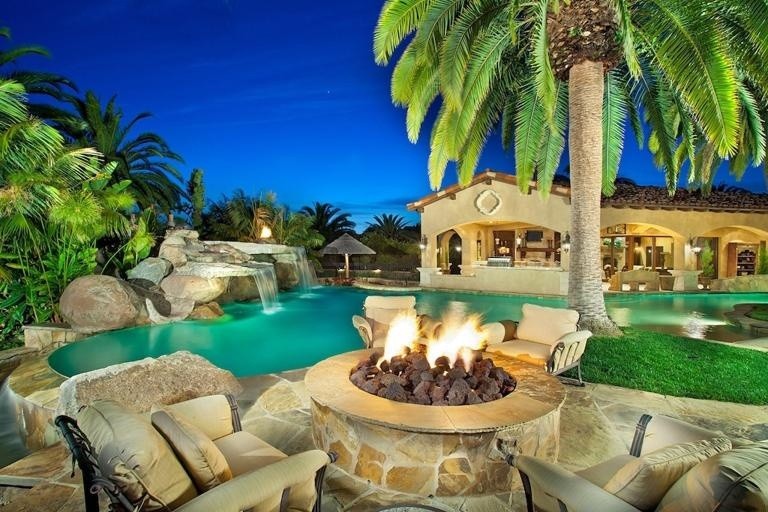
[658,275,675,290]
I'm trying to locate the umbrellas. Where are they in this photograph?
[318,232,376,278]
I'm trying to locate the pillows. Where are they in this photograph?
[371,307,417,348]
[149,401,233,497]
[601,434,734,512]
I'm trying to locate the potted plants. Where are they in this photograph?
[697,239,717,286]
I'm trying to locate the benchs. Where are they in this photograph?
[607,269,660,293]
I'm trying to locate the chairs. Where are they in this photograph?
[353,295,439,354]
[503,411,767,512]
[477,302,589,385]
[50,388,337,511]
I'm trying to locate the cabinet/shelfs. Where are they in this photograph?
[728,242,760,277]
